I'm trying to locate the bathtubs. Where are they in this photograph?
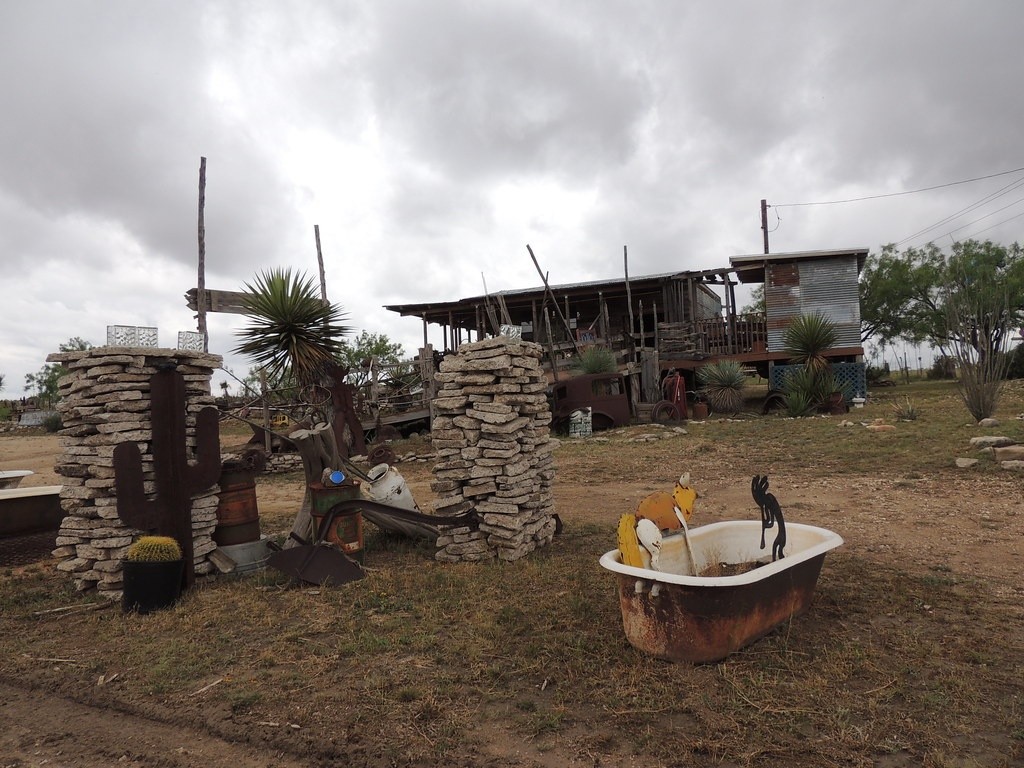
[0,470,34,489]
[599,520,844,666]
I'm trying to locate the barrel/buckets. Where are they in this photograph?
[213,461,260,543]
[309,480,364,570]
[365,463,422,515]
[569,406,593,439]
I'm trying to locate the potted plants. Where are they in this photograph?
[383,362,422,411]
[121,536,183,613]
